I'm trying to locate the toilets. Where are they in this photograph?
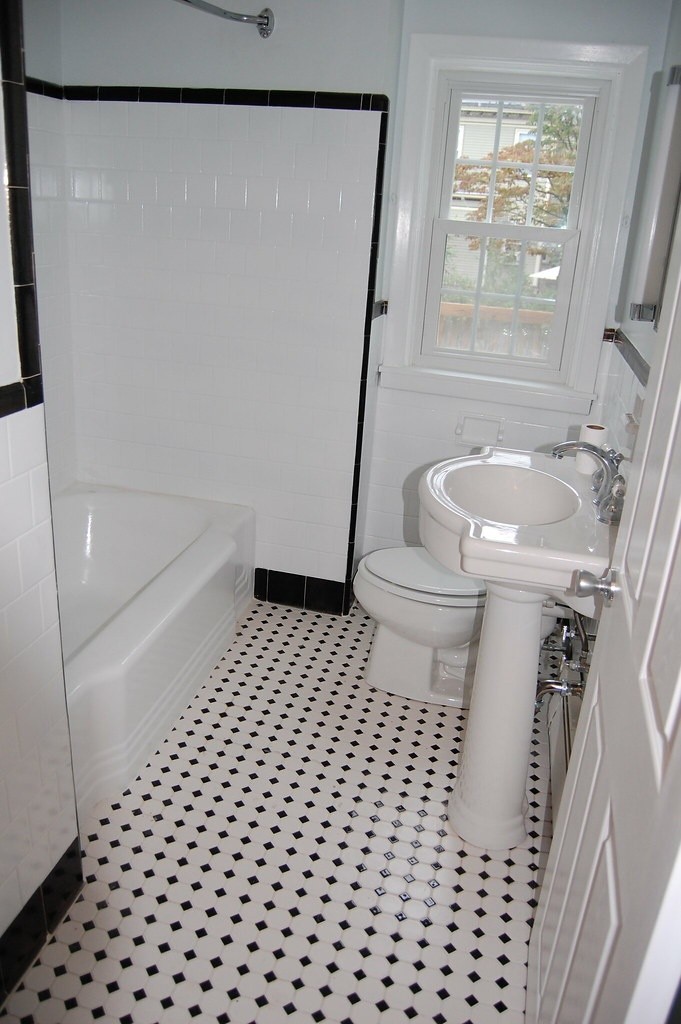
[352,545,488,710]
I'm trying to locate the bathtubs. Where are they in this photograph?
[48,474,258,842]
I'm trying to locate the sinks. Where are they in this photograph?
[415,442,614,627]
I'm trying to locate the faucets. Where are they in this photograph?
[550,437,623,505]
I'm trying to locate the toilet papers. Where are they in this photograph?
[575,419,609,477]
